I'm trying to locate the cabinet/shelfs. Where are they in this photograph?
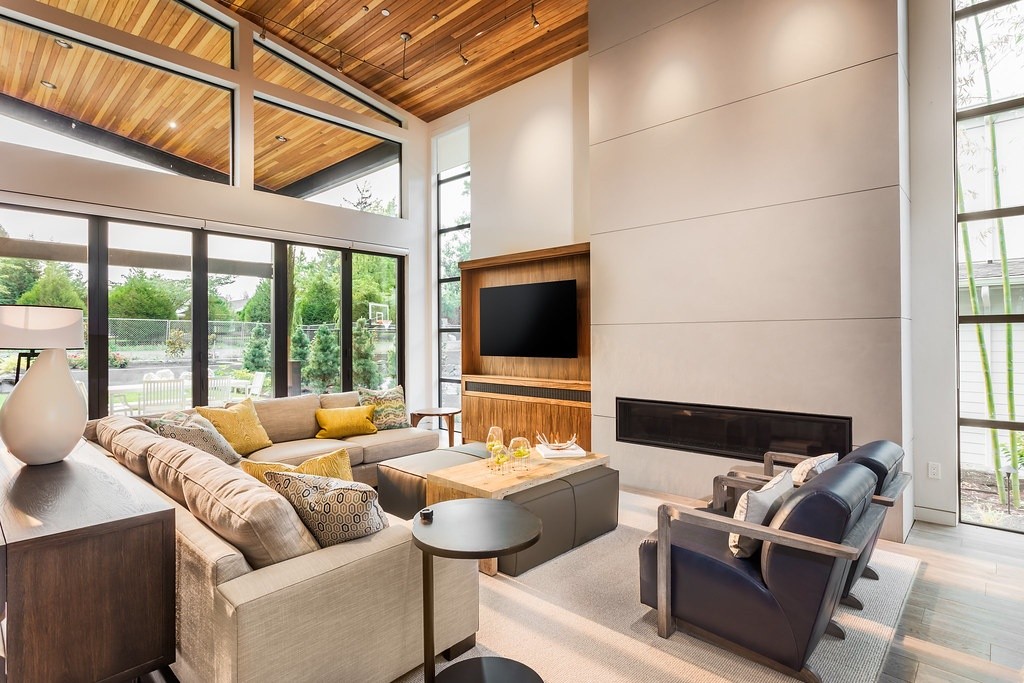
[0,435,176,683]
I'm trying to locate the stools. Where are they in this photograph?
[376,440,619,577]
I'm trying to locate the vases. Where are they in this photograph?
[0,348,87,465]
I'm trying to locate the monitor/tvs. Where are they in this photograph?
[479,278,578,359]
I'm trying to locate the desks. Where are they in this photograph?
[229,380,250,397]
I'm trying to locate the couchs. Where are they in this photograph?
[83,391,439,683]
[639,440,911,683]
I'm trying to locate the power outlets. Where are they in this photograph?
[928,462,941,479]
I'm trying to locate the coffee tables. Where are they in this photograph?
[412,498,544,683]
[410,407,461,447]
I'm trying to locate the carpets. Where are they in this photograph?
[381,488,921,683]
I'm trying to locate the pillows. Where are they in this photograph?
[195,397,273,456]
[239,448,354,485]
[791,452,838,482]
[728,470,793,558]
[357,385,413,430]
[315,404,377,438]
[141,409,190,433]
[158,413,243,464]
[263,471,389,547]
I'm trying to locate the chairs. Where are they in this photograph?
[248,372,266,396]
[76,381,134,416]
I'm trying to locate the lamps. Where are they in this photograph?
[337,62,344,73]
[260,28,266,40]
[458,44,468,65]
[531,4,540,28]
[0,304,85,385]
